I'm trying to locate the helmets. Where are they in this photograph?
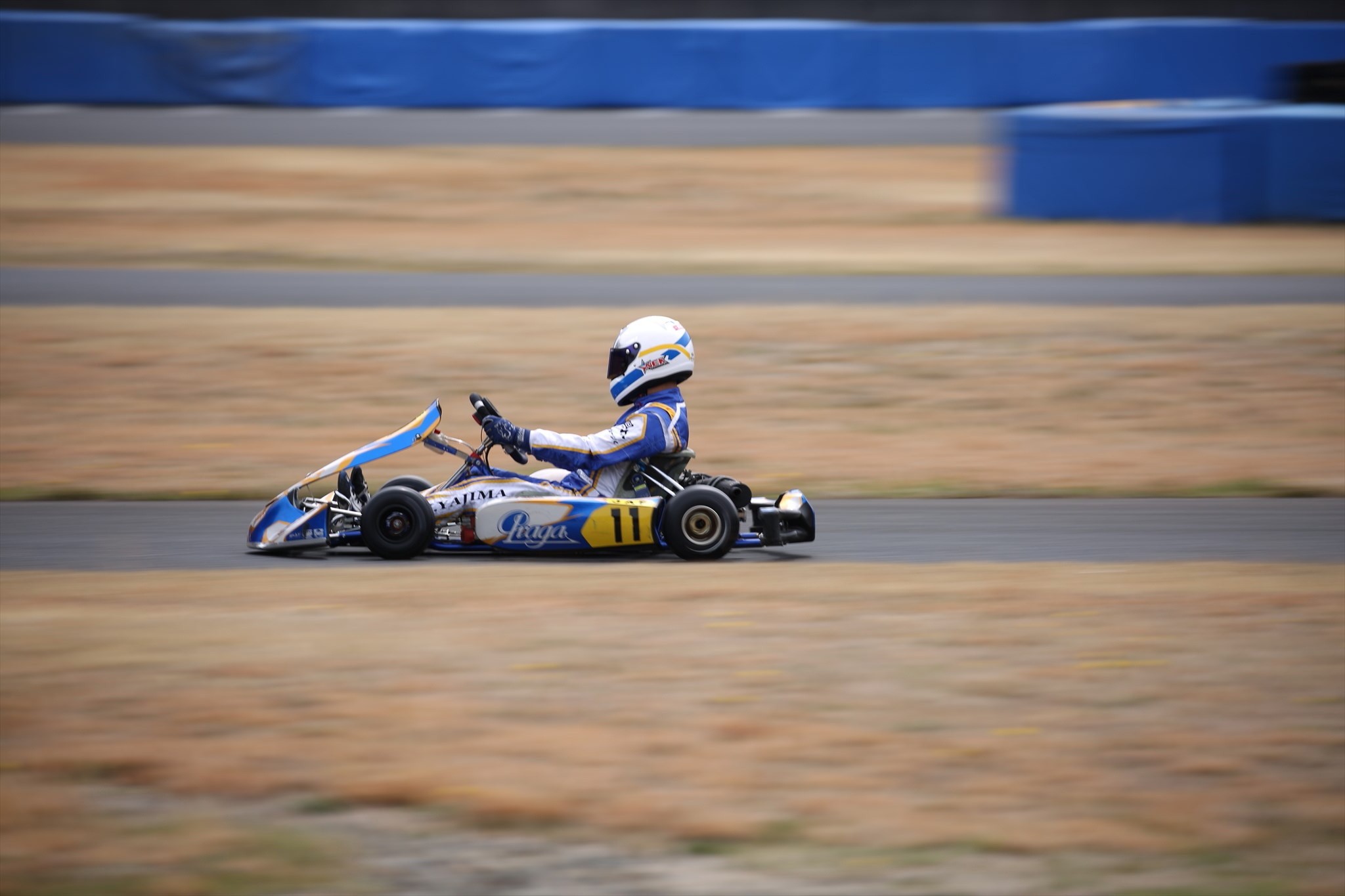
[607,315,694,406]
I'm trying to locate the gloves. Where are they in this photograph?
[482,415,517,445]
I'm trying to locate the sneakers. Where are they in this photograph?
[337,471,363,528]
[349,466,371,503]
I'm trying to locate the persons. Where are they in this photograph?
[337,316,696,527]
